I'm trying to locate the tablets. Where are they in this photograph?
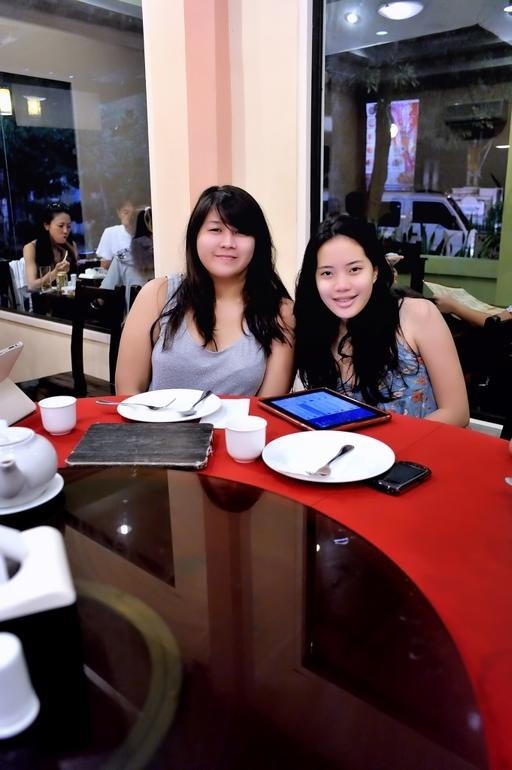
[256,387,392,434]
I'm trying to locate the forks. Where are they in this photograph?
[306,444,354,477]
[96,399,177,411]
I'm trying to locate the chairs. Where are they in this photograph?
[129,286,142,311]
[15,287,125,402]
[384,242,427,293]
[479,317,512,417]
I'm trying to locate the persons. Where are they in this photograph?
[23,210,79,310]
[294,215,470,427]
[113,185,297,399]
[436,295,512,325]
[96,199,137,270]
[99,209,151,310]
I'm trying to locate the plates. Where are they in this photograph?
[117,388,221,423]
[261,429,396,483]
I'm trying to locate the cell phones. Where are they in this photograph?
[373,461,431,494]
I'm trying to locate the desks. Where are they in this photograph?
[32,294,103,319]
[0,395,512,770]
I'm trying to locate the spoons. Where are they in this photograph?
[177,389,212,416]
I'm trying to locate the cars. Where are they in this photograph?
[377,192,476,257]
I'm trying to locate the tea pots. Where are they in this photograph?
[0,418,58,508]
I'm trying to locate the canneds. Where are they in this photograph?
[56,271,68,292]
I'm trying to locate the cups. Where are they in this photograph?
[40,265,51,292]
[0,632,40,737]
[225,415,268,463]
[37,396,77,436]
